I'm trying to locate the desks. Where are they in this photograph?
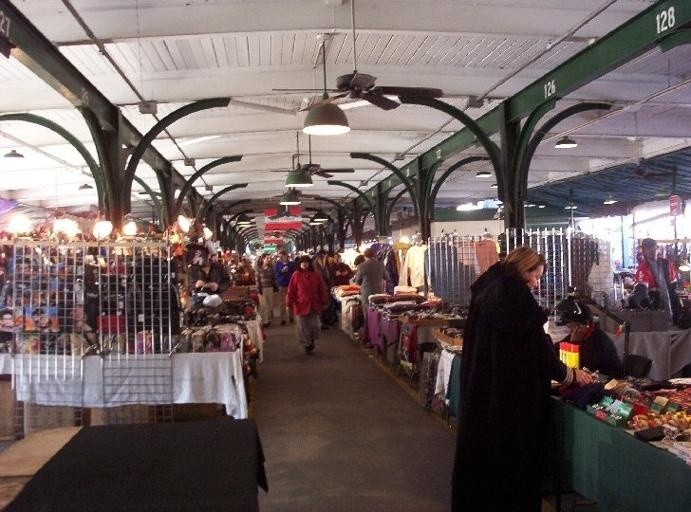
[330,282,690,512]
[0,274,270,509]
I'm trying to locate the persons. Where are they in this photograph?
[0,254,65,332]
[353,249,390,344]
[450,246,590,510]
[555,302,621,379]
[285,255,328,352]
[256,251,355,328]
[636,239,677,308]
[186,262,229,298]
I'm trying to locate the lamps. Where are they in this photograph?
[4,139,23,159]
[474,138,618,209]
[0,214,213,241]
[284,132,313,188]
[301,33,351,138]
[78,182,95,192]
[279,155,303,207]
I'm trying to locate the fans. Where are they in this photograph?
[268,191,300,245]
[274,186,320,201]
[269,96,355,179]
[273,0,443,114]
